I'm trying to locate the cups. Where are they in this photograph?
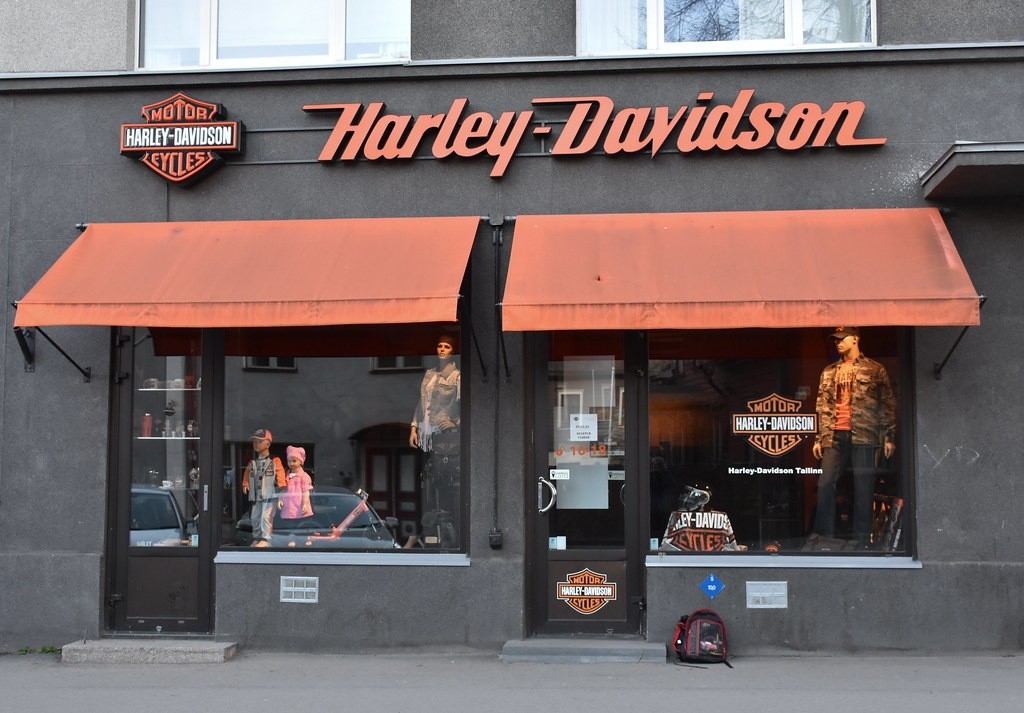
[162,480,174,488]
[142,378,158,389]
[170,379,185,389]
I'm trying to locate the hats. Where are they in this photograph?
[249,428,272,443]
[828,326,860,338]
[286,446,306,465]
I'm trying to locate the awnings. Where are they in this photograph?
[501,207,982,331]
[13,215,483,327]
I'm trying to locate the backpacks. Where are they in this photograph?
[672,608,734,669]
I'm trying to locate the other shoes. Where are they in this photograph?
[800,532,832,552]
[853,543,872,551]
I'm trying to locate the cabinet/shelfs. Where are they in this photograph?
[132,389,200,491]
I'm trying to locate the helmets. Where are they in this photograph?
[674,481,712,512]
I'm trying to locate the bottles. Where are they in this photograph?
[141,412,151,438]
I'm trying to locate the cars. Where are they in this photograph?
[129,478,201,546]
[235,487,400,549]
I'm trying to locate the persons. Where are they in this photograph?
[277,445,314,547]
[813,327,896,551]
[403,336,460,549]
[241,428,287,547]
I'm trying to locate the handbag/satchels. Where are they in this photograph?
[421,507,458,548]
